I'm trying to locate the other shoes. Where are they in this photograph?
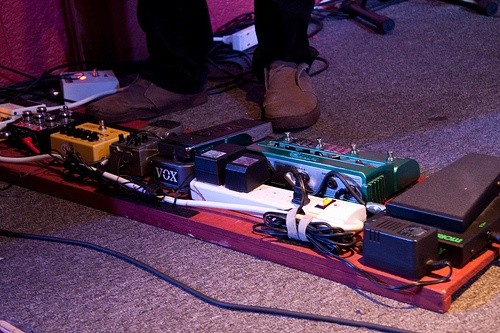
[85,78,210,125]
[263,60,321,131]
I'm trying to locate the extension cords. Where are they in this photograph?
[189,176,367,234]
[232,24,258,51]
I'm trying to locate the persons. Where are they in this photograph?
[88,0,320,129]
